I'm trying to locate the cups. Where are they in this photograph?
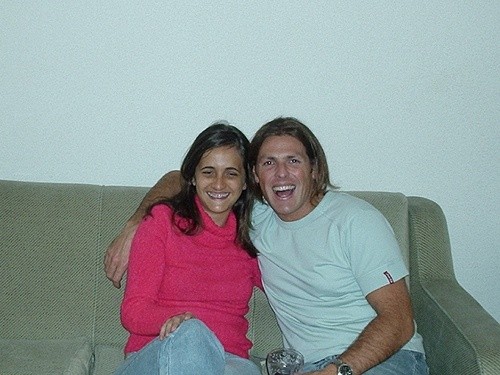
[265,347,305,375]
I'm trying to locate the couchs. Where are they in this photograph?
[0,180,500,375]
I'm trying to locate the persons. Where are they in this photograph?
[104,116,429,375]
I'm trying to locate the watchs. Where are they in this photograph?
[324,358,353,375]
